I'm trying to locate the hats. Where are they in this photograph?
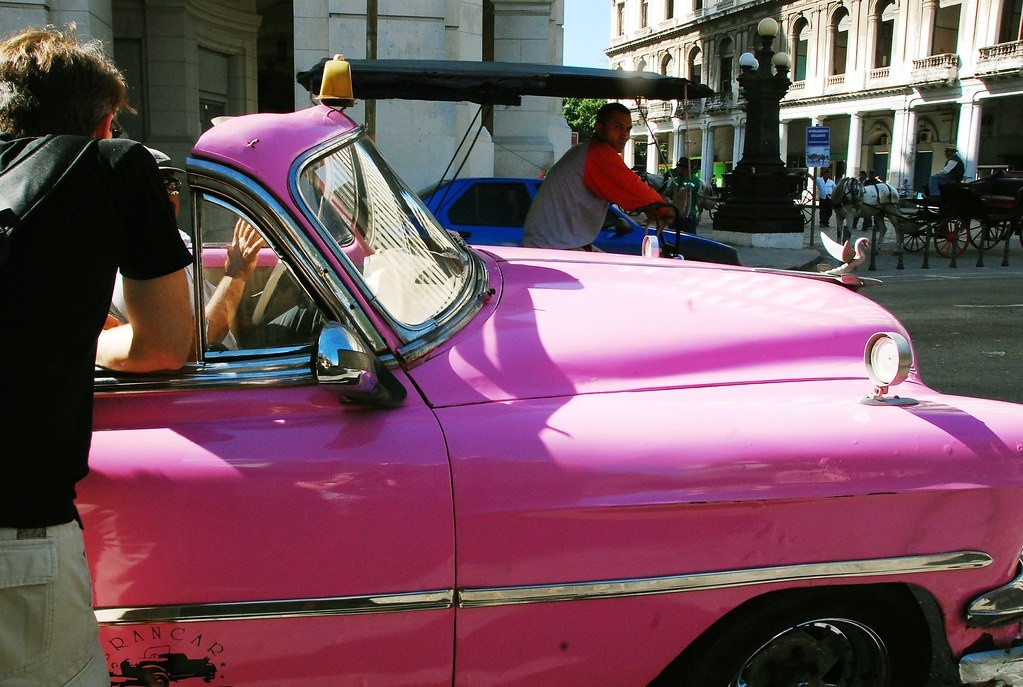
[144,145,186,173]
[945,143,956,150]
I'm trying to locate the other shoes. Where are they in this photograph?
[844,225,856,229]
[826,224,829,227]
[862,226,867,231]
[820,223,825,227]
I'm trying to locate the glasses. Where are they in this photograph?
[945,151,948,153]
[162,178,181,197]
[96,112,122,139]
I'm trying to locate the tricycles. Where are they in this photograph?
[292,55,716,261]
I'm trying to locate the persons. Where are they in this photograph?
[0,25,194,687]
[669,144,964,229]
[121,145,264,353]
[522,102,668,252]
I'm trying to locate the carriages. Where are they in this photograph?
[635,169,814,226]
[829,171,1023,259]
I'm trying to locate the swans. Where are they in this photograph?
[820,230,870,268]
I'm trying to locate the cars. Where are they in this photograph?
[411,176,744,267]
[77,50,1023,686]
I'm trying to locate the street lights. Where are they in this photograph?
[712,16,804,234]
